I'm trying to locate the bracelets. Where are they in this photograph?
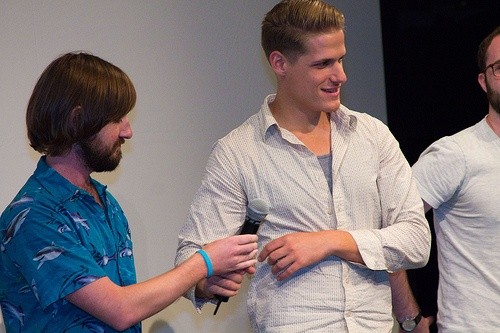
[196,250,213,278]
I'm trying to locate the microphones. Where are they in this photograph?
[213,198,270,316]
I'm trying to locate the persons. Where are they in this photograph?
[0,51,258,333]
[389,32,500,333]
[175,0,430,333]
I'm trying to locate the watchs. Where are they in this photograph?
[396,312,423,331]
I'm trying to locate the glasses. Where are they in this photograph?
[484,59,500,80]
